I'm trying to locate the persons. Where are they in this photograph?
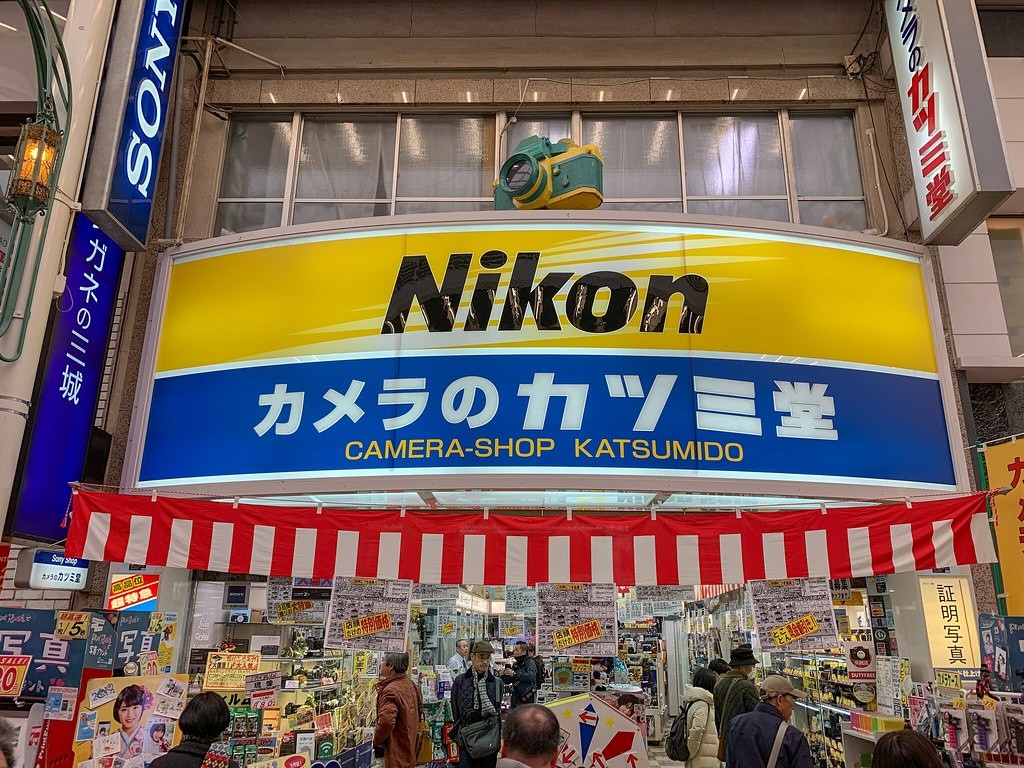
[713,648,762,734]
[617,694,638,717]
[373,652,424,768]
[985,634,992,652]
[871,729,945,768]
[110,685,153,759]
[148,691,239,768]
[98,727,106,738]
[995,654,1006,674]
[447,639,468,668]
[144,723,171,753]
[158,701,183,715]
[725,675,814,768]
[0,719,18,768]
[451,641,504,768]
[684,668,724,768]
[501,641,544,710]
[709,658,729,682]
[497,704,560,768]
[167,681,183,698]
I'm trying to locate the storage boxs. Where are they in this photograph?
[220,609,267,655]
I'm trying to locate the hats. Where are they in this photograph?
[728,647,760,665]
[759,675,807,700]
[472,641,495,654]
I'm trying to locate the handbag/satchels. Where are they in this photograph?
[717,735,727,762]
[461,716,500,758]
[417,711,431,763]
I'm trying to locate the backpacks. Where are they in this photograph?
[664,699,709,762]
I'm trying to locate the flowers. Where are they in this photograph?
[294,636,308,655]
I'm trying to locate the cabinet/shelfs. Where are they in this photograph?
[260,621,344,706]
[751,650,913,763]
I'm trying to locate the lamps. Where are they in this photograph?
[9,95,66,226]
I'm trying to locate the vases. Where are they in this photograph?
[292,650,305,661]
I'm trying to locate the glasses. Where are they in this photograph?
[475,654,492,661]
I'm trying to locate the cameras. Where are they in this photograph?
[850,646,869,660]
[261,645,279,655]
[231,613,248,623]
[492,135,605,209]
[281,665,338,716]
